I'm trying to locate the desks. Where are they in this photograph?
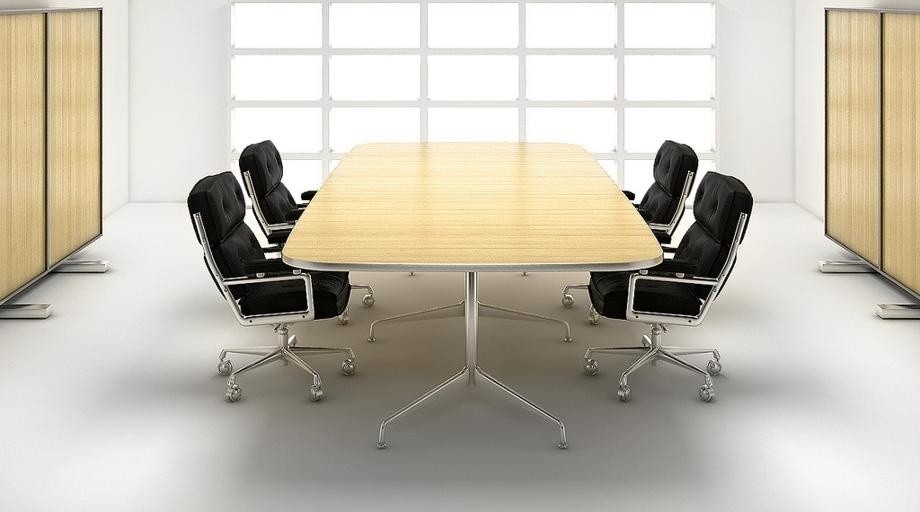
[280,141,664,450]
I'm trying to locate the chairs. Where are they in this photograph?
[186,171,358,401]
[239,140,374,325]
[562,140,698,323]
[583,171,754,401]
